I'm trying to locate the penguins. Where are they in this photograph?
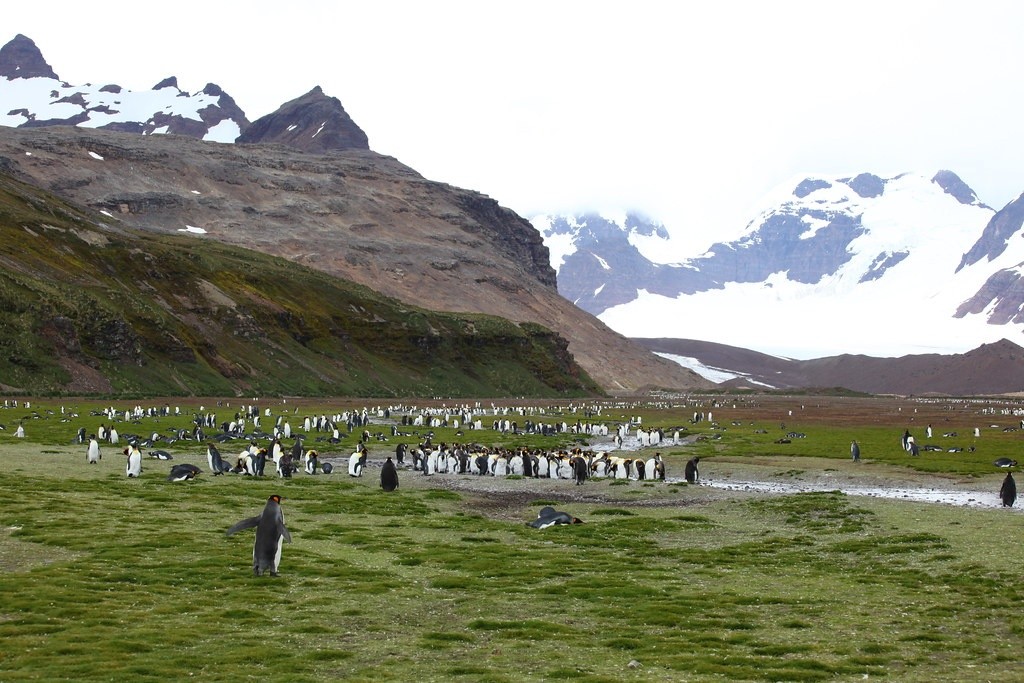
[0,396,1024,531]
[226,495,291,577]
